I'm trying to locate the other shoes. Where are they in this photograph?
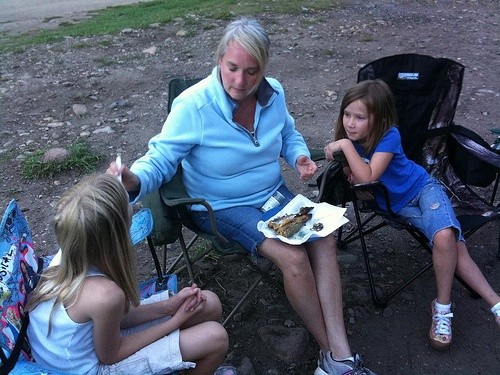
[208,366,237,375]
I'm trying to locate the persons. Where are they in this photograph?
[323,79,500,348]
[29,172,229,375]
[104,19,376,375]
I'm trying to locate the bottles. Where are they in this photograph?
[260,190,285,214]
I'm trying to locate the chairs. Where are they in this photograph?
[337,52,500,310]
[143,78,342,331]
[0,196,179,375]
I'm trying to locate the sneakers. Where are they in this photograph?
[314,349,377,375]
[490,301,500,326]
[430,298,454,346]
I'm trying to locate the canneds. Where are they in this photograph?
[259,191,285,212]
